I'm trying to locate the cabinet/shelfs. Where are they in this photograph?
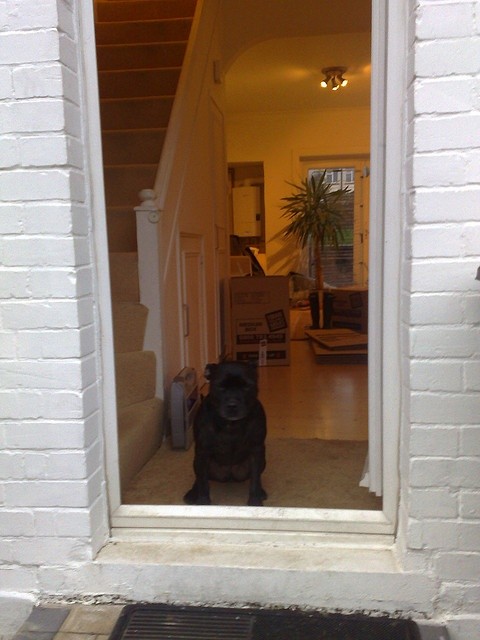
[176,230,210,391]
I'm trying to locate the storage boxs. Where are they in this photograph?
[221,274,291,368]
[330,286,368,333]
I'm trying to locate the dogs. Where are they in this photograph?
[183,362,267,505]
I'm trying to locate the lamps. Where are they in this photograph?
[319,67,350,92]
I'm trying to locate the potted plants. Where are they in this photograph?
[279,172,350,327]
